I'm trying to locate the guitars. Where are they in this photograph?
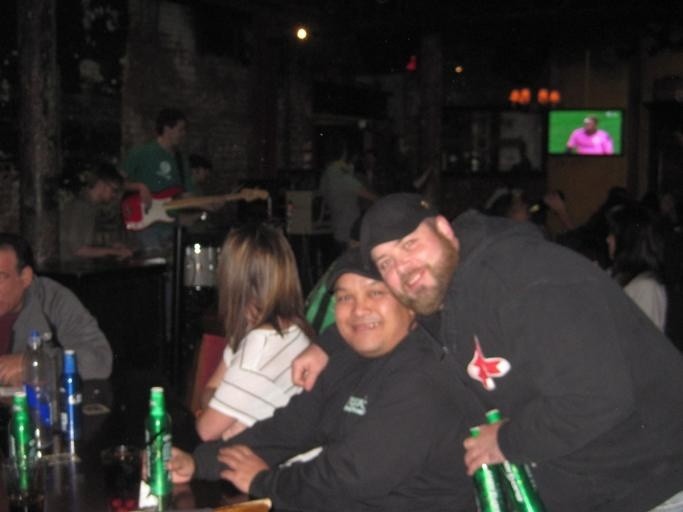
[121,186,267,230]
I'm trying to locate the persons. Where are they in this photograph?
[194,220,323,465]
[122,111,224,218]
[321,139,681,332]
[0,233,113,388]
[289,191,683,512]
[566,117,611,155]
[141,249,488,511]
[51,141,224,270]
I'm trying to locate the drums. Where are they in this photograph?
[180,244,221,288]
[124,247,168,267]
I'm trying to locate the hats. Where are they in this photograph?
[326,247,384,295]
[359,193,440,255]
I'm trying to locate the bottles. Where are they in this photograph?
[6,392,40,476]
[140,387,175,498]
[464,407,546,512]
[57,348,85,442]
[22,331,55,448]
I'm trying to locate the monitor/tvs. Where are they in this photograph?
[545,105,624,161]
